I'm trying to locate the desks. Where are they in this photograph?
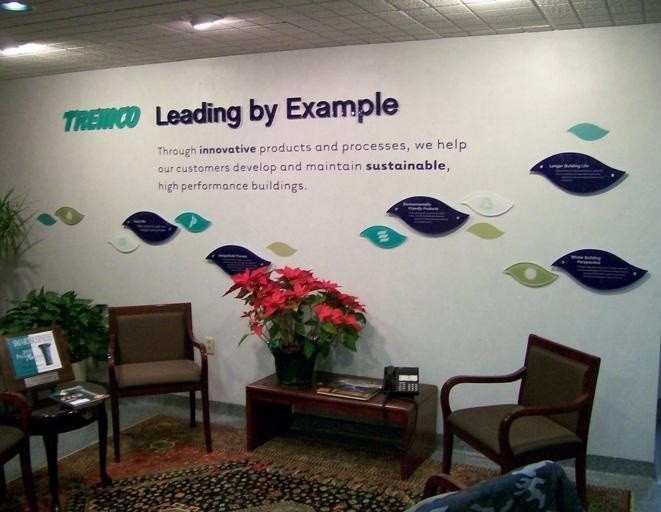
[246,370,439,481]
[29,394,113,511]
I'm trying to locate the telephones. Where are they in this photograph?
[383,365,420,399]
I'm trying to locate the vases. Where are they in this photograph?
[270,343,324,386]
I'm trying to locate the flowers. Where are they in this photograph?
[220,262,369,359]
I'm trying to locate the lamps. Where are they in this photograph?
[179,10,226,31]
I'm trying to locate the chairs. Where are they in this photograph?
[400,459,587,511]
[1,387,45,511]
[106,301,214,463]
[440,333,602,502]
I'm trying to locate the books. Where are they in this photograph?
[48,385,110,408]
[316,377,383,400]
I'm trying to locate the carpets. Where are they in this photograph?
[1,410,636,511]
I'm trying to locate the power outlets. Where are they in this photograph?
[205,337,216,354]
